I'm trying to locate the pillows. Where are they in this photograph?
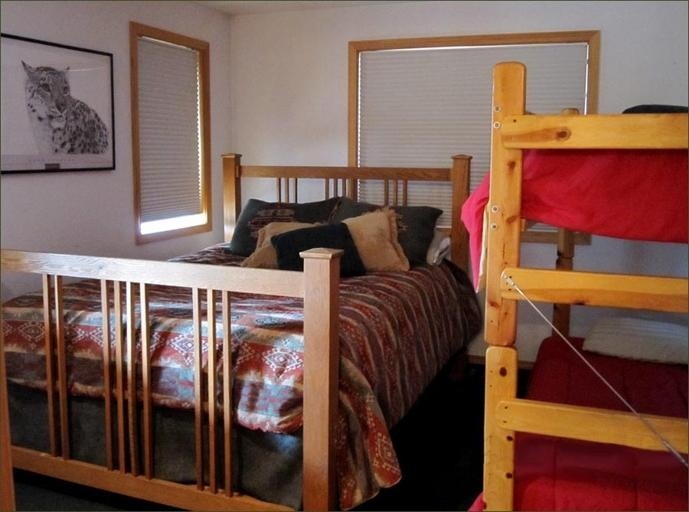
[582,313,688,367]
[617,103,689,113]
[330,196,444,271]
[230,195,339,257]
[240,207,411,274]
[270,221,366,284]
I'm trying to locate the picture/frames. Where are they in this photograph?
[0,32,117,176]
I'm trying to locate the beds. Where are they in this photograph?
[1,151,472,510]
[466,50,689,508]
